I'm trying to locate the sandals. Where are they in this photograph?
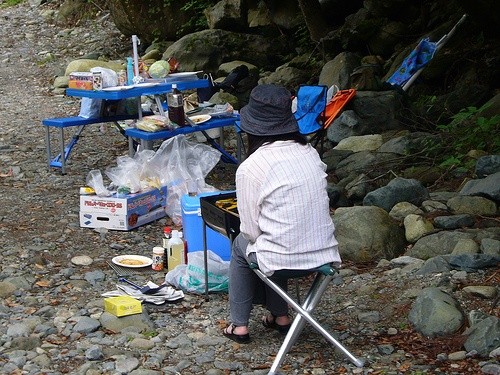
[262,316,291,335]
[223,324,250,344]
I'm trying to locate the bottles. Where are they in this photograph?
[127,57,134,85]
[119,70,126,85]
[152,226,188,273]
[93,72,102,90]
[167,83,185,126]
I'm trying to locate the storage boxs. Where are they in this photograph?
[181,190,236,263]
[69,71,94,91]
[80,185,168,219]
[79,199,166,230]
[103,296,143,317]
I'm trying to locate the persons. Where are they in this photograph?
[223,85,342,343]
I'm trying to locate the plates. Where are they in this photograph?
[185,114,212,125]
[112,255,153,268]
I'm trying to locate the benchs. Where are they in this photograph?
[124,109,242,168]
[42,102,170,177]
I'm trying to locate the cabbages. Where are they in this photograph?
[148,59,171,78]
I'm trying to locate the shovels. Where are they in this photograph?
[119,277,166,294]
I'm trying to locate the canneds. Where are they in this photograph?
[119,70,127,85]
[152,246,164,270]
[93,71,103,90]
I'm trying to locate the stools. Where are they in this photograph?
[247,260,365,375]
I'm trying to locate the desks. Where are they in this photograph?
[65,77,210,160]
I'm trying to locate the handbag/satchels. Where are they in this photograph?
[197,72,220,101]
[222,64,249,93]
[80,68,118,119]
[179,249,231,293]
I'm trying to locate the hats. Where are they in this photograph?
[239,84,300,136]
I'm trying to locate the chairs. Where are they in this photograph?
[290,84,329,161]
